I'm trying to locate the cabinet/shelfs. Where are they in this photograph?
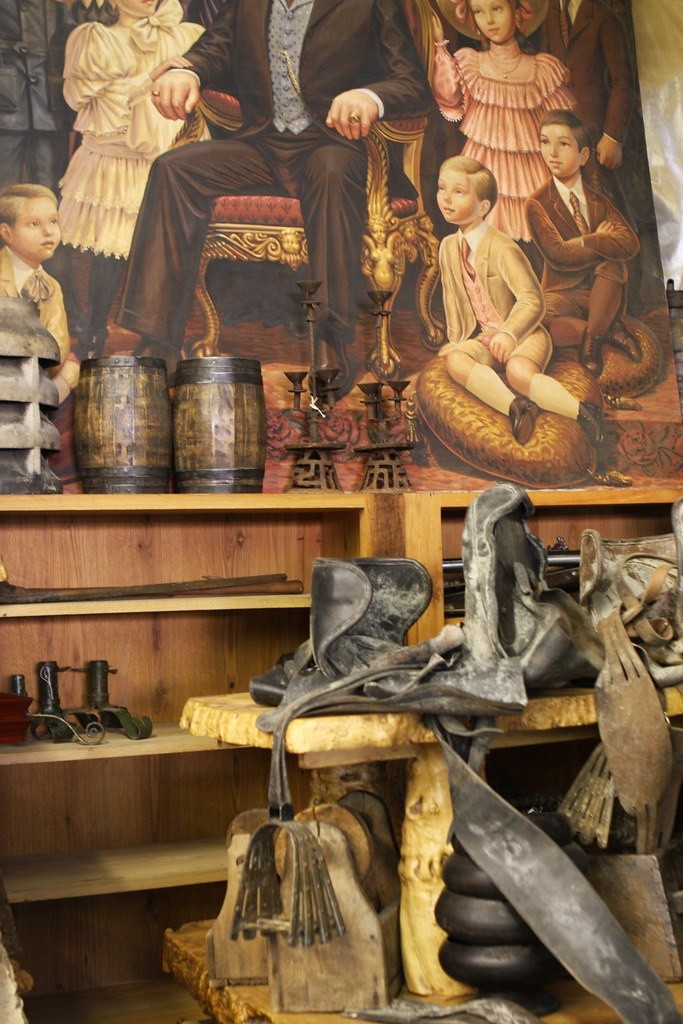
[158,691,683,1024]
[0,486,683,1024]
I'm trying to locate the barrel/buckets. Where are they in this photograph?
[74,355,171,494]
[171,358,268,494]
